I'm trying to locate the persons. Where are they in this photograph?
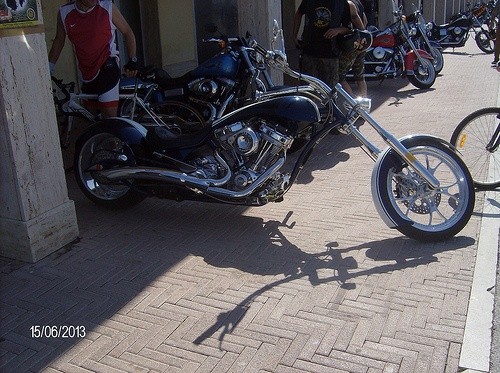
[489,0,500,68]
[294,0,353,84]
[47,0,138,119]
[338,0,368,96]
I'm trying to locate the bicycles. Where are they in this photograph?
[449,106,500,190]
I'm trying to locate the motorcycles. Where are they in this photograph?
[342,1,500,90]
[70,18,475,244]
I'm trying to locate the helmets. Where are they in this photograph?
[348,28,373,52]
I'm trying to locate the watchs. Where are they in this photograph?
[132,57,137,62]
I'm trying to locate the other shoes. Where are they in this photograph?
[492,59,499,64]
